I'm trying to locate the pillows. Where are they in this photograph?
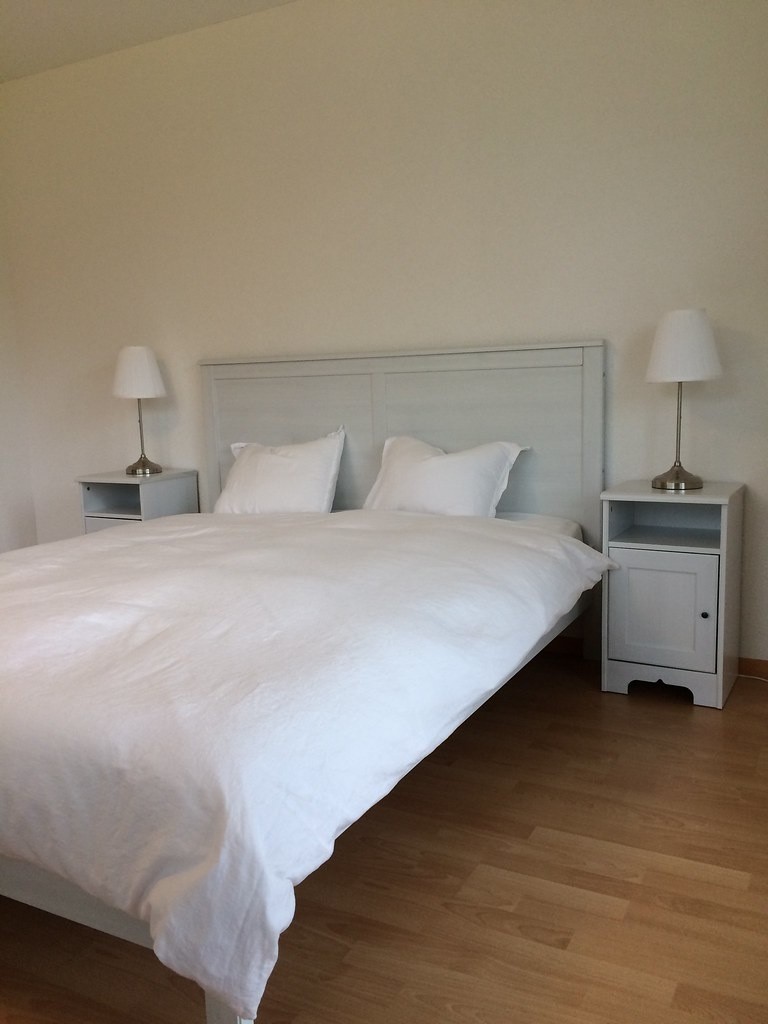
[209,424,346,514]
[361,435,531,518]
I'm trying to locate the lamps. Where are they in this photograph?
[112,346,167,475]
[645,308,723,491]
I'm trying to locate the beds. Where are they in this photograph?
[0,338,620,1024]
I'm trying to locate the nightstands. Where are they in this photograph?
[599,479,747,710]
[75,469,200,534]
